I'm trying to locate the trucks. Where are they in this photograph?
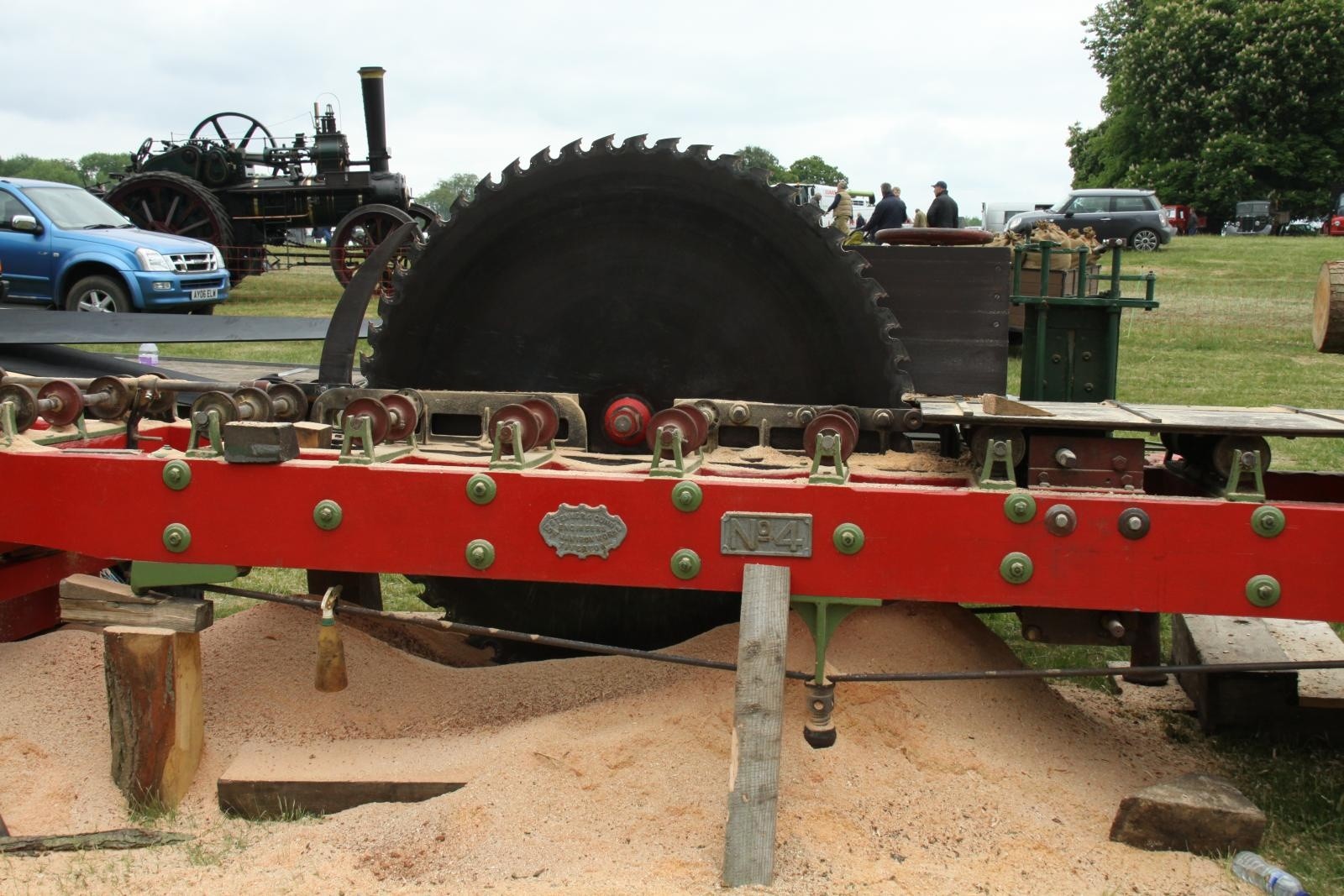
[772,183,915,230]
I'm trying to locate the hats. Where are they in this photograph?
[932,182,947,190]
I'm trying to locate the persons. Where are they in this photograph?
[926,181,959,246]
[913,208,927,228]
[313,225,332,250]
[1183,207,1198,235]
[824,182,854,235]
[811,193,829,226]
[856,183,907,246]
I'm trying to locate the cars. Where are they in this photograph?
[0,176,231,315]
[1221,192,1344,235]
[965,189,1207,252]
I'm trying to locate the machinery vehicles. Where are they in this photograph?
[83,66,439,296]
